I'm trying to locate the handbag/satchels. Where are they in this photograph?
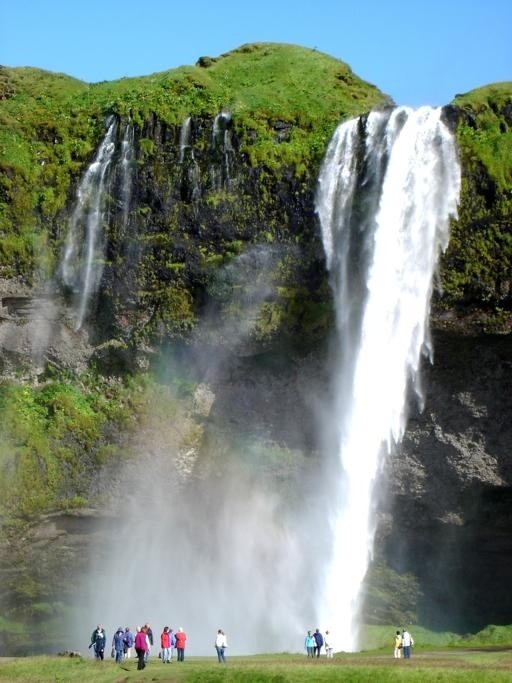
[111,648,115,657]
[397,643,402,648]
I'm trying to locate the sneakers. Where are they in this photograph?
[163,662,165,663]
[167,660,172,663]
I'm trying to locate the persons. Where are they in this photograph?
[160,626,176,664]
[215,628,228,664]
[401,628,414,659]
[304,630,317,659]
[394,630,402,660]
[89,622,153,670]
[175,627,186,661]
[313,628,323,657]
[324,631,334,659]
